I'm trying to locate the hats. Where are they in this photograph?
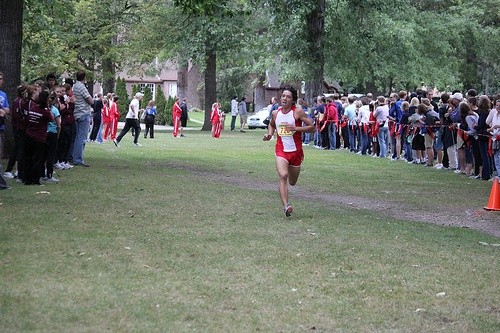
[241,97,247,101]
[134,92,145,99]
[233,95,238,99]
[450,92,463,101]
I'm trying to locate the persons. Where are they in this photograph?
[112,91,145,147]
[230,95,238,132]
[172,97,188,138]
[210,101,222,140]
[143,100,157,139]
[239,97,248,132]
[0,71,123,193]
[262,85,315,218]
[264,88,500,186]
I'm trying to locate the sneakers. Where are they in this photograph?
[283,203,294,218]
[113,138,119,147]
[134,142,142,148]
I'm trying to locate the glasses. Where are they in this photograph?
[390,97,394,99]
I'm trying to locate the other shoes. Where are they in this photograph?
[4,170,18,179]
[56,159,90,170]
[454,169,500,183]
[50,176,59,182]
[313,143,452,169]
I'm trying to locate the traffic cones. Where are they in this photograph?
[484,176,500,211]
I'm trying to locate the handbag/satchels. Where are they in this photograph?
[379,120,386,127]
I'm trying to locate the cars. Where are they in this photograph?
[247,108,271,129]
